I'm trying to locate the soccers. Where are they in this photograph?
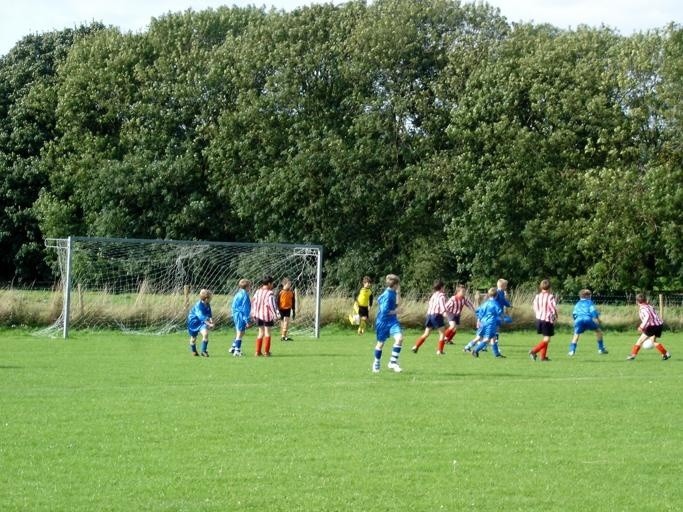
[643,339,654,350]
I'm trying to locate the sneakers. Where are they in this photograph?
[541,356,551,360]
[464,346,472,352]
[663,353,670,359]
[449,339,454,344]
[598,349,608,354]
[256,352,262,356]
[495,353,507,358]
[482,347,488,351]
[373,362,380,371]
[388,363,401,372]
[201,352,209,357]
[412,346,418,353]
[192,351,199,355]
[281,337,286,340]
[529,350,537,361]
[234,350,242,356]
[624,354,635,359]
[568,351,575,355]
[264,351,272,356]
[472,351,479,358]
[437,351,447,355]
[285,337,293,341]
[229,348,235,352]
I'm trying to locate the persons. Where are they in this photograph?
[494,279,513,352]
[354,276,373,336]
[472,287,507,359]
[412,279,449,355]
[626,294,671,360]
[229,279,251,356]
[372,274,404,373]
[528,280,558,361]
[187,289,215,358]
[568,289,608,356]
[462,296,488,353]
[275,278,296,341]
[444,286,475,345]
[251,276,278,357]
